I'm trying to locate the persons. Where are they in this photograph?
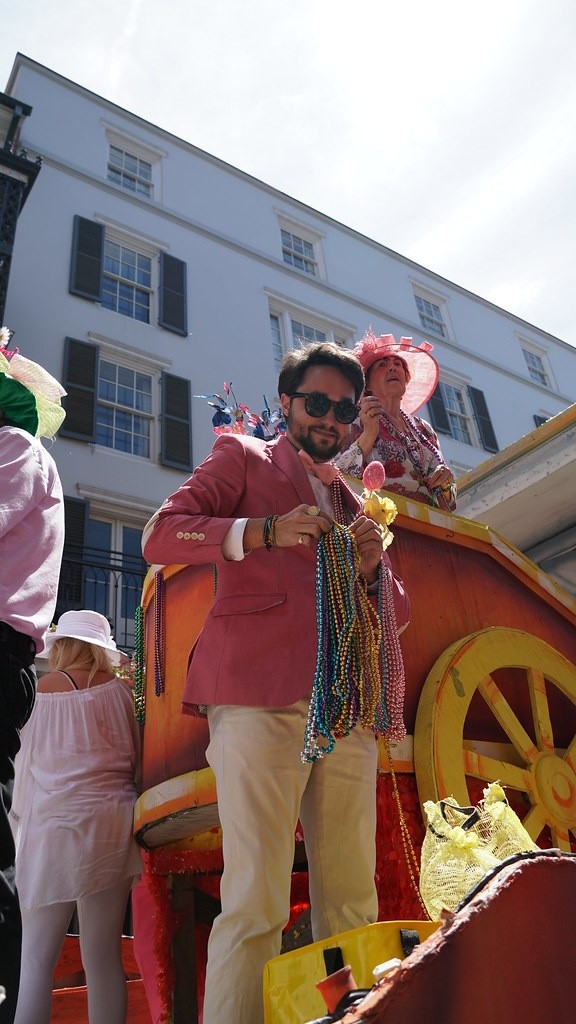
[333,334,457,512]
[0,324,65,1024]
[7,610,144,1024]
[140,344,411,1024]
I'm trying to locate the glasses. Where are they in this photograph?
[291,392,361,425]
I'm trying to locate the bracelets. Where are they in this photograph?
[263,515,279,550]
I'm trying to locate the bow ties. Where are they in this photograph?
[299,448,338,486]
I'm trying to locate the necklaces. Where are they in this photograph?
[380,410,445,493]
[213,563,217,597]
[432,484,452,512]
[330,461,364,526]
[133,607,144,723]
[368,557,407,742]
[154,572,165,696]
[300,527,382,764]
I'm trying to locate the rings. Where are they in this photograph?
[359,400,364,404]
[307,506,322,516]
[446,479,451,484]
[437,468,444,474]
[298,535,305,544]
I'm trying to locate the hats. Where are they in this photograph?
[0,327,68,439]
[354,327,439,415]
[35,609,128,668]
